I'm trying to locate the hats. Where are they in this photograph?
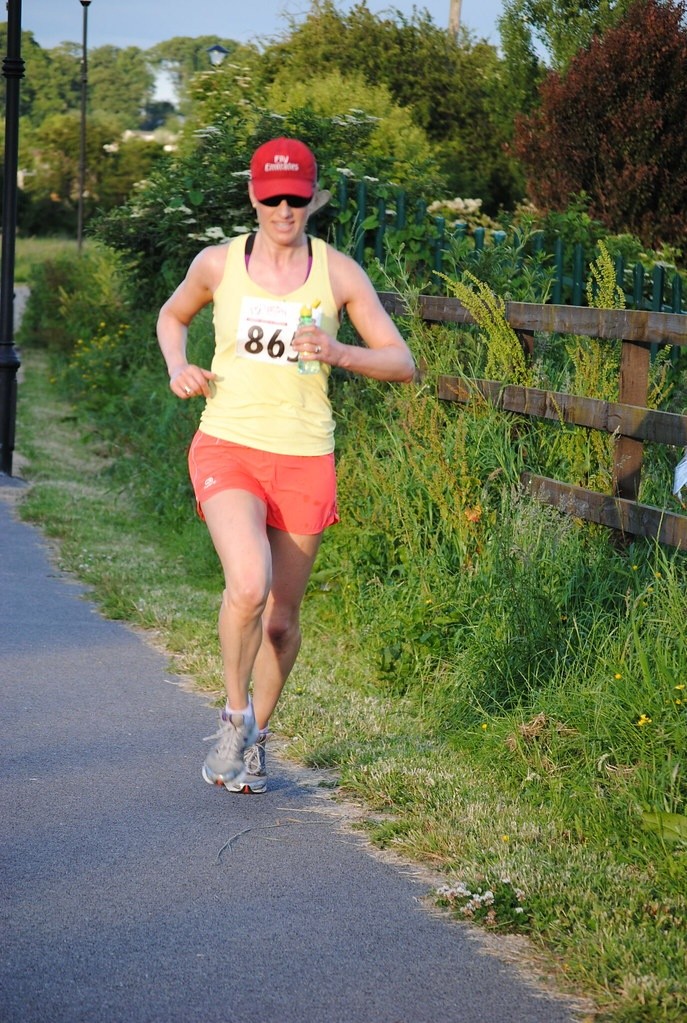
[251,136,315,201]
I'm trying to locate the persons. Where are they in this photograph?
[152,132,418,797]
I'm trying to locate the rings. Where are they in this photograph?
[315,345,321,355]
[184,386,192,395]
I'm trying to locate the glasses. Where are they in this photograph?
[261,194,312,207]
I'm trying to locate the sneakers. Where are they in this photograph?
[226,732,272,793]
[202,700,260,786]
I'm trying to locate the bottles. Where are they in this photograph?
[298,298,322,374]
